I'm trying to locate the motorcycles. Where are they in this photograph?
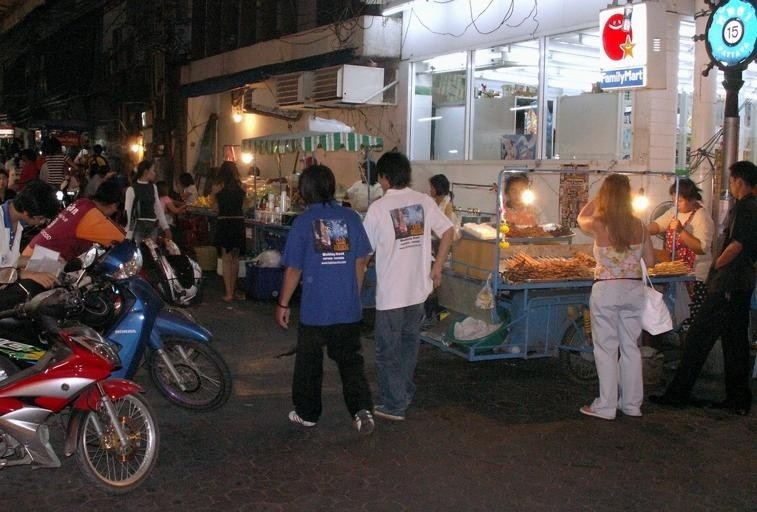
[1,246,161,495]
[1,236,233,413]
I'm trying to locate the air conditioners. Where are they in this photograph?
[311,64,383,102]
[271,71,330,112]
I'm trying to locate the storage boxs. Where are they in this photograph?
[217,257,249,279]
[194,246,217,271]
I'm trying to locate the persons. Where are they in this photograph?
[18,180,127,273]
[0,179,61,314]
[503,175,547,225]
[275,163,379,436]
[1,129,248,302]
[647,160,756,419]
[343,160,383,220]
[577,173,657,423]
[360,151,455,423]
[429,174,462,267]
[640,177,718,364]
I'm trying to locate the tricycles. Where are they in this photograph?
[419,166,695,387]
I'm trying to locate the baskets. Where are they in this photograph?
[148,278,206,308]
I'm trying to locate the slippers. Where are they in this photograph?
[580,405,616,420]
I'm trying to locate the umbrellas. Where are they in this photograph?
[192,114,218,192]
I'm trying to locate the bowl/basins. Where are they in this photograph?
[444,305,512,355]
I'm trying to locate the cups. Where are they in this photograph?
[252,189,287,226]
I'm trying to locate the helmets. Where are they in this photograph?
[160,255,202,304]
[139,238,181,274]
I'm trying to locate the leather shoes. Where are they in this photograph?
[648,394,686,408]
[721,400,748,415]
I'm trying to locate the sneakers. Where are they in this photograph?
[77,431,100,446]
[289,411,316,427]
[374,405,406,420]
[355,409,375,435]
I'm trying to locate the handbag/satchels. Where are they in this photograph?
[640,257,673,336]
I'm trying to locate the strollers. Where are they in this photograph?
[140,235,207,308]
